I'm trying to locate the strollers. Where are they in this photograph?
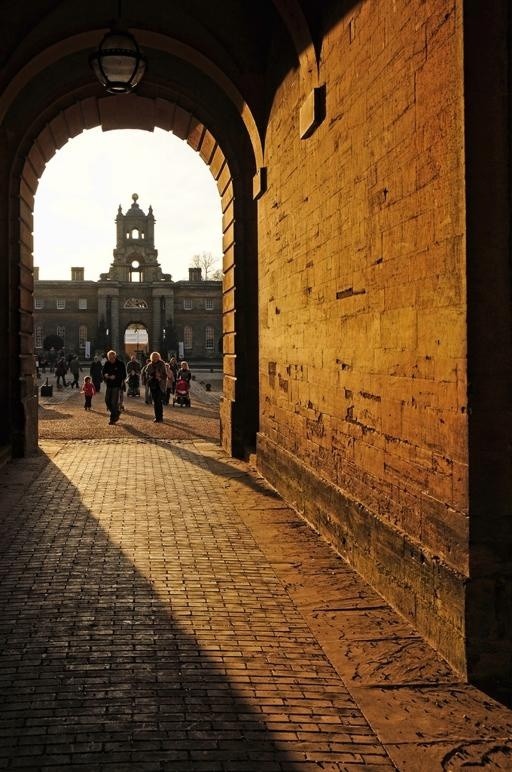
[173,379,190,407]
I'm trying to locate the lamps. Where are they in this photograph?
[88,0,149,95]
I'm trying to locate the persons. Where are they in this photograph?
[32,343,192,425]
